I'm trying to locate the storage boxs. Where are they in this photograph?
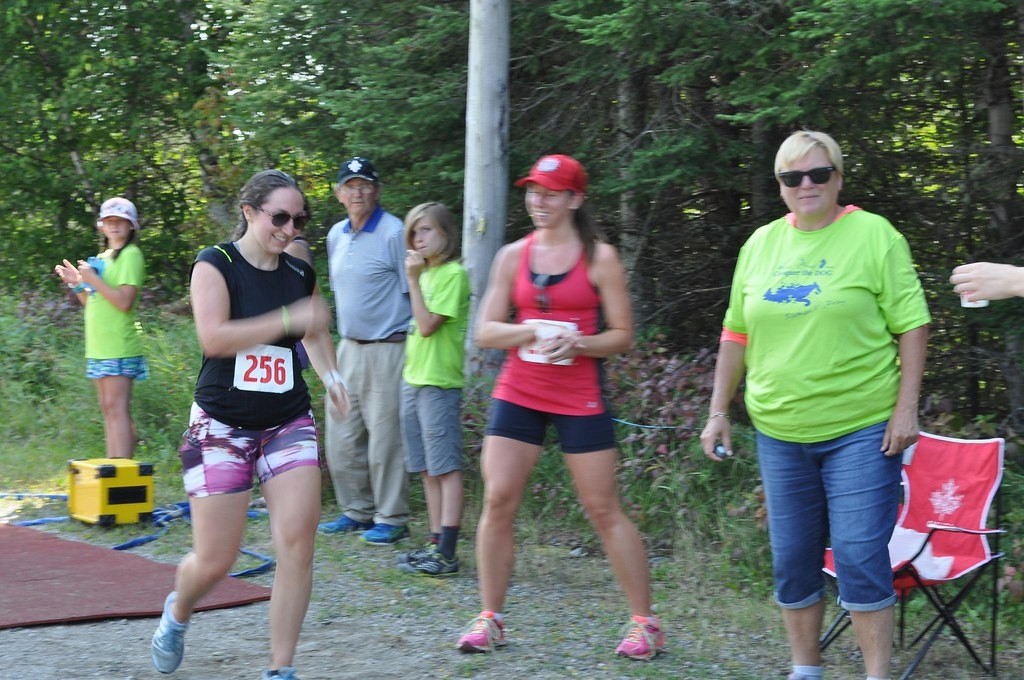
[68,457,154,527]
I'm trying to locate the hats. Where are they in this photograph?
[338,158,379,183]
[515,155,586,193]
[100,198,139,230]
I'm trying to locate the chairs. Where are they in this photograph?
[818,430,1009,680]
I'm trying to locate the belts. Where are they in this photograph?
[357,332,407,344]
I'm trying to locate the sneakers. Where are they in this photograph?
[400,540,440,567]
[615,613,664,661]
[455,610,507,652]
[262,666,299,680]
[406,552,461,576]
[360,523,411,545]
[152,591,188,674]
[315,515,374,535]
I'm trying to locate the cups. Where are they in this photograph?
[960,290,989,308]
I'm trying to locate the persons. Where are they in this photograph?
[151,168,353,680]
[396,203,470,578]
[699,132,930,680]
[948,262,1024,302]
[454,153,665,660]
[55,198,149,458]
[324,158,412,546]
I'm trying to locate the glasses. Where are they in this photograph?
[778,167,836,187]
[532,274,550,313]
[343,184,375,194]
[255,207,309,229]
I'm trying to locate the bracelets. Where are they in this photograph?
[322,371,343,390]
[705,412,729,421]
[282,306,290,339]
[68,283,83,292]
